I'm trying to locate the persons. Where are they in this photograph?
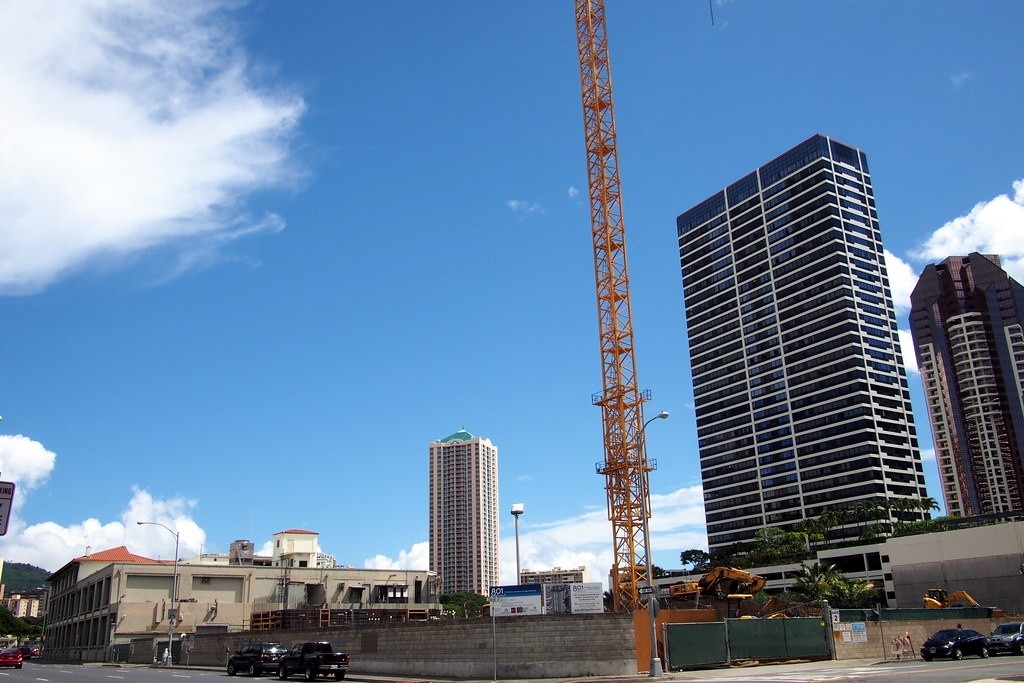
[162,647,168,665]
[957,624,961,630]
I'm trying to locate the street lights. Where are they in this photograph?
[136,521,180,667]
[637,411,669,677]
[510,503,524,585]
[37,611,47,636]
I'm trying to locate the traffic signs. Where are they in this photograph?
[637,586,653,595]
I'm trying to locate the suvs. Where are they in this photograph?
[227,642,288,677]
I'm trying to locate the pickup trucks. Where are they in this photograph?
[276,641,349,682]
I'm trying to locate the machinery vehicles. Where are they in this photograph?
[922,588,981,608]
[668,566,767,604]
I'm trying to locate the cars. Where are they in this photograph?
[0,646,43,669]
[987,621,1024,657]
[920,627,990,662]
